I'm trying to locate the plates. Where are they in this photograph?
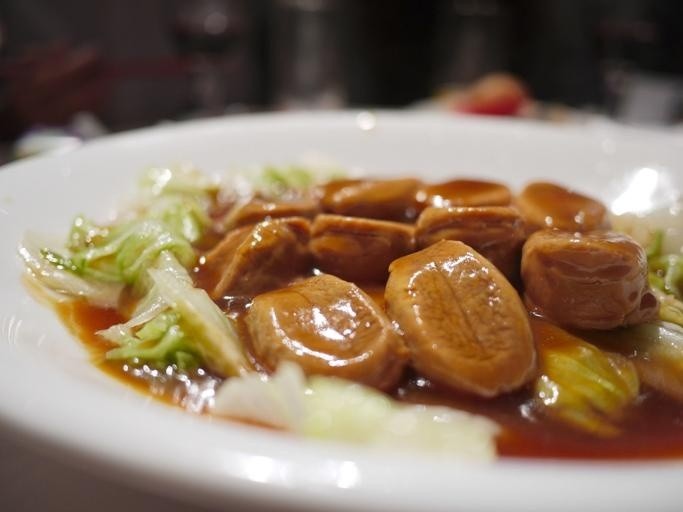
[1,107,681,511]
